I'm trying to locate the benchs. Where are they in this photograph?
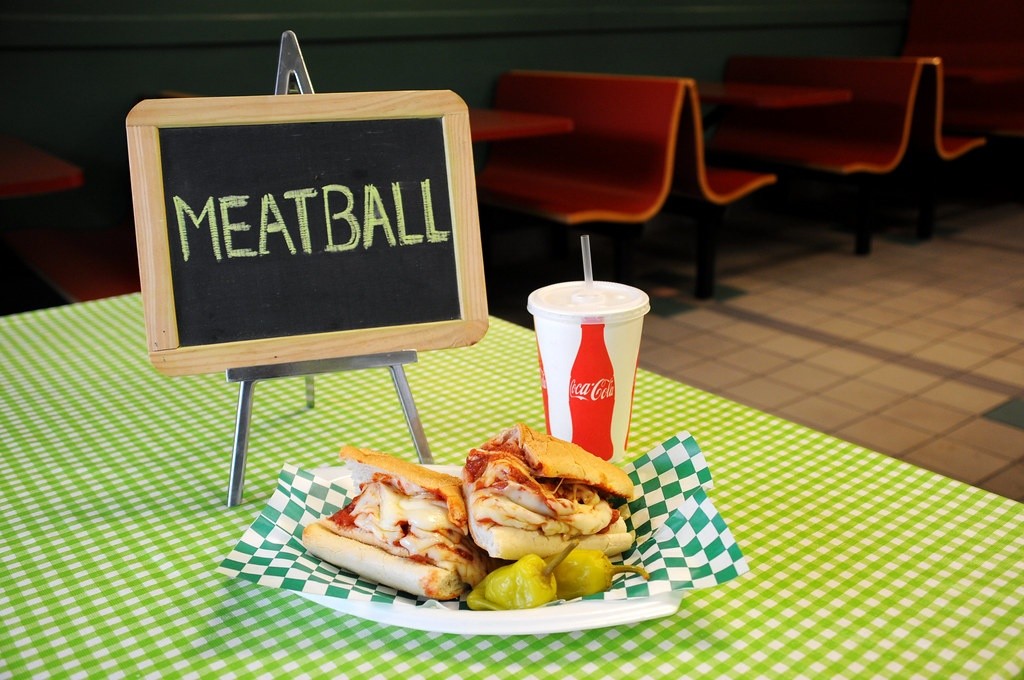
[663,75,778,300]
[474,67,685,281]
[6,94,176,303]
[701,55,927,255]
[897,39,1024,206]
[904,55,988,241]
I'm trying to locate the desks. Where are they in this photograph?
[668,81,855,242]
[945,66,1024,95]
[1,291,1024,680]
[0,130,84,199]
[470,108,575,142]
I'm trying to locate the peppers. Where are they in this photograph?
[466,537,580,610]
[542,548,652,600]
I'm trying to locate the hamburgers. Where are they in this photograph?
[300,444,510,600]
[460,422,635,559]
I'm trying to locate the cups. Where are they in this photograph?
[526,281,650,465]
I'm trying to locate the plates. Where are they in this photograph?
[285,591,683,636]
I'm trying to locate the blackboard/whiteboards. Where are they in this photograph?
[126,89,488,378]
